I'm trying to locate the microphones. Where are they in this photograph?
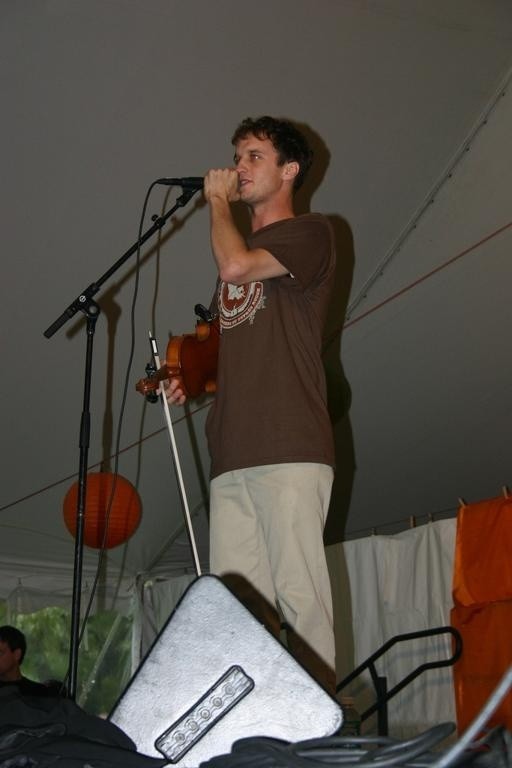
[155,170,208,195]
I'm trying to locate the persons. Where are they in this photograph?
[156,115,338,700]
[0,625,40,684]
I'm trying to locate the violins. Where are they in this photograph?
[135,314,218,405]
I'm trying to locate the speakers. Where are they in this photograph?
[104,572,346,768]
